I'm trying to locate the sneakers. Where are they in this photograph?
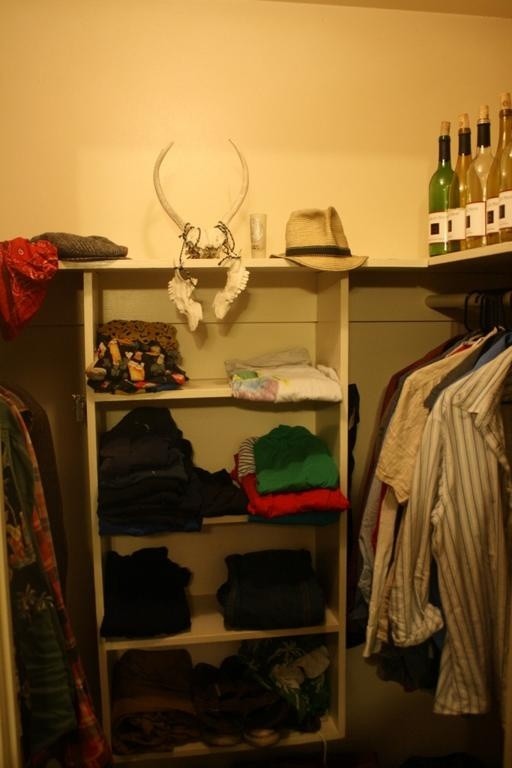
[221,648,281,748]
[189,661,241,747]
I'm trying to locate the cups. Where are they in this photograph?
[249,213,267,258]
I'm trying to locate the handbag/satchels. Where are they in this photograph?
[27,232,131,263]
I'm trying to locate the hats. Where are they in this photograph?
[269,205,371,275]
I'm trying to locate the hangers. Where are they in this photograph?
[464,289,511,405]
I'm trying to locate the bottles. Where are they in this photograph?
[427,91,512,256]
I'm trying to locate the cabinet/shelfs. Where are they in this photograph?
[77,251,354,765]
